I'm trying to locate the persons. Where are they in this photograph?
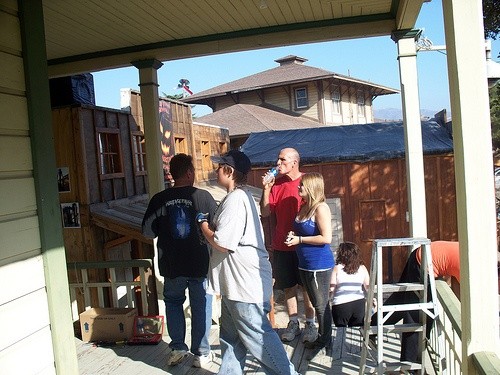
[284,172,335,360]
[141,153,219,368]
[260,148,319,343]
[196,150,301,375]
[318,242,376,346]
[360,241,500,375]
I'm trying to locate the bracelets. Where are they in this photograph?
[299,236,302,244]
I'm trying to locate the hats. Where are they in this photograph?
[209,150,252,174]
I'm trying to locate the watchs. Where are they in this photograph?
[198,220,209,225]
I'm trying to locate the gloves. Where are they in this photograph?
[195,212,209,226]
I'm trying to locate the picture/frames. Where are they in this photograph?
[56,167,71,193]
[61,203,82,229]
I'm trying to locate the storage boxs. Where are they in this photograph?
[127,316,164,346]
[49,73,95,107]
[79,307,138,342]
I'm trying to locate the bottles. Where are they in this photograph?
[261,166,279,185]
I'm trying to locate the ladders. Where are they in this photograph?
[360,237,449,375]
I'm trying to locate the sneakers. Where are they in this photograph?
[193,350,216,368]
[168,349,189,366]
[305,337,332,349]
[281,320,300,341]
[301,323,318,343]
[359,327,378,353]
